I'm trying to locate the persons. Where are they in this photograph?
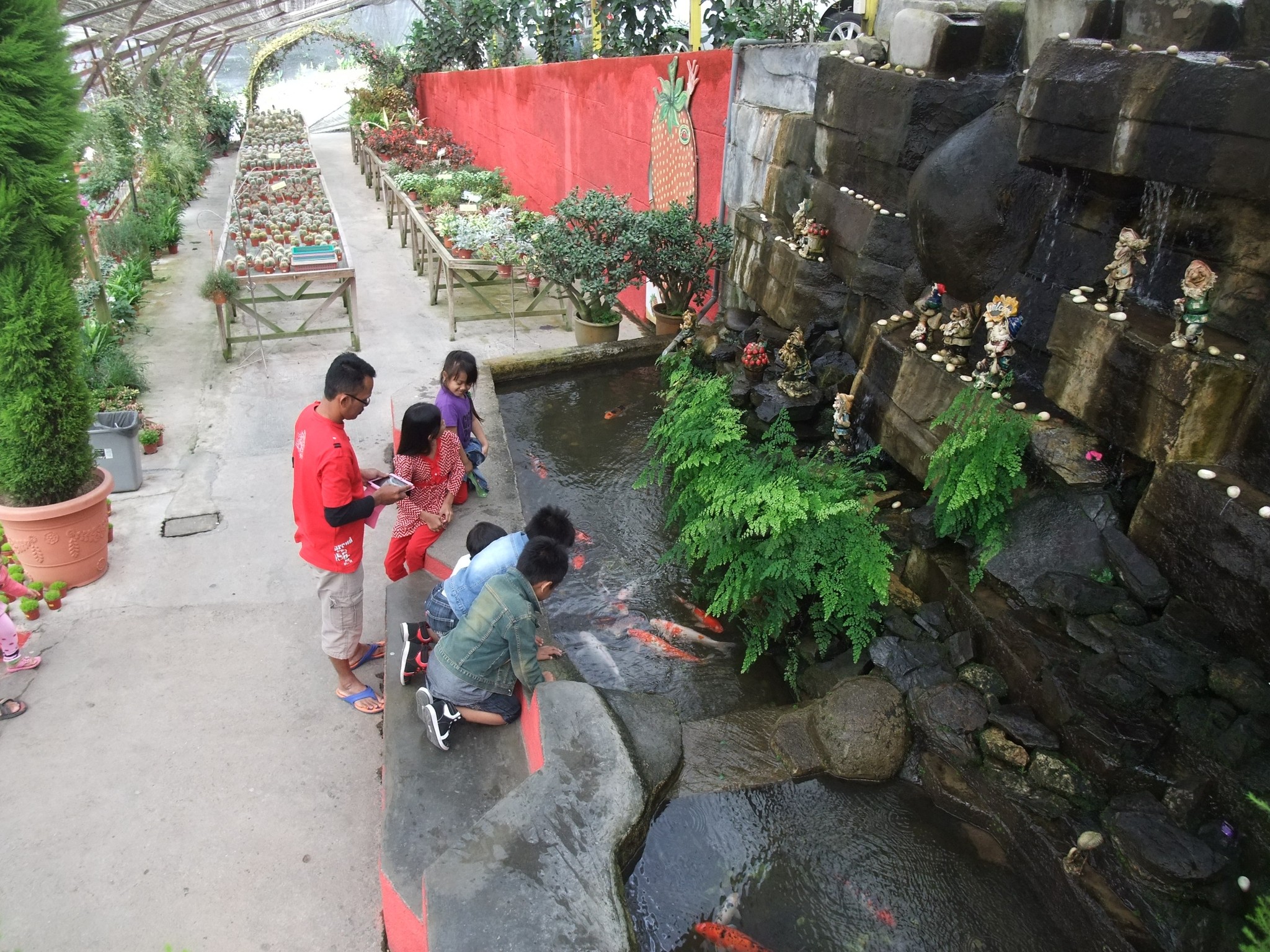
[384,403,465,582]
[1097,227,1148,311]
[798,217,816,248]
[1172,260,1218,349]
[435,350,489,506]
[920,283,946,311]
[830,393,855,451]
[777,326,810,397]
[401,504,575,686]
[447,522,507,577]
[787,198,813,243]
[415,535,568,751]
[292,351,412,714]
[910,319,927,343]
[976,316,1023,388]
[931,304,974,366]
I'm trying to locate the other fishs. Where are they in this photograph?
[566,546,588,570]
[590,559,616,602]
[624,626,717,666]
[575,628,628,687]
[583,572,662,640]
[524,440,549,479]
[829,870,898,929]
[648,617,742,660]
[692,920,771,952]
[573,527,593,546]
[712,890,743,952]
[603,396,647,421]
[667,587,725,634]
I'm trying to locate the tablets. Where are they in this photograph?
[368,473,414,491]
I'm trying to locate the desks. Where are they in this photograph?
[211,174,362,364]
[408,204,575,341]
[349,122,396,202]
[377,164,431,270]
[234,125,323,176]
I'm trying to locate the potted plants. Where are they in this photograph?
[0,0,745,588]
[27,581,45,601]
[0,595,9,606]
[19,599,41,621]
[43,589,62,610]
[48,580,67,598]
[0,590,16,603]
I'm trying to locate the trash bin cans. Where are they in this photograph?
[87,411,144,492]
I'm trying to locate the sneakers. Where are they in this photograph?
[400,641,431,686]
[400,621,432,645]
[422,698,462,751]
[415,686,441,722]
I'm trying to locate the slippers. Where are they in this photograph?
[350,643,385,670]
[336,684,384,714]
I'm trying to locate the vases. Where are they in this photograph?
[743,363,767,382]
[806,233,825,251]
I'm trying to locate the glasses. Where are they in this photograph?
[345,394,370,407]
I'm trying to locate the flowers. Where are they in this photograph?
[741,340,769,367]
[808,223,829,237]
